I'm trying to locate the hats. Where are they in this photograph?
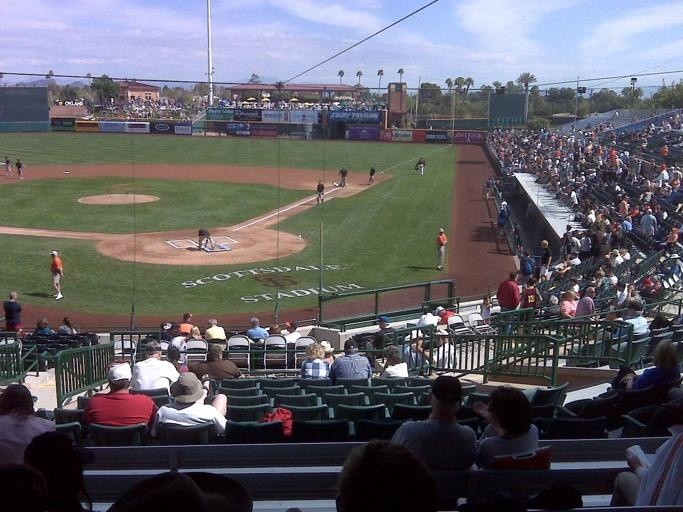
[170,372,204,403]
[405,329,430,341]
[628,302,644,311]
[438,228,445,233]
[50,250,59,256]
[670,254,681,259]
[321,341,334,353]
[434,306,444,314]
[107,361,133,384]
[432,376,462,404]
[610,249,619,256]
[344,338,359,349]
[376,316,391,324]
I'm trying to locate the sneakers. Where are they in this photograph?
[53,291,64,300]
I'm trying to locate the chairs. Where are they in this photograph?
[18,311,682,446]
[517,116,683,310]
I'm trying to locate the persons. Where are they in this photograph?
[54,97,387,123]
[339,165,347,187]
[316,180,324,205]
[197,230,215,250]
[484,109,682,431]
[49,250,64,300]
[4,156,24,180]
[1,291,682,512]
[437,227,448,270]
[416,157,425,176]
[368,164,375,185]
